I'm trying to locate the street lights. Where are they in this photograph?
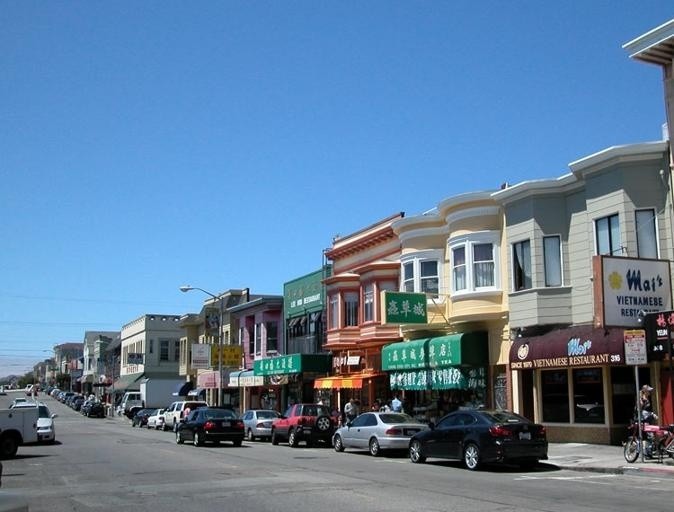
[178,287,224,407]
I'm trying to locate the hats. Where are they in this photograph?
[642,384,653,391]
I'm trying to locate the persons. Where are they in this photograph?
[337,397,360,430]
[316,396,329,416]
[183,404,192,421]
[634,384,660,459]
[371,392,405,414]
[88,393,96,400]
[288,398,295,411]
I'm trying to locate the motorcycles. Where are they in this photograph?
[623,410,674,463]
